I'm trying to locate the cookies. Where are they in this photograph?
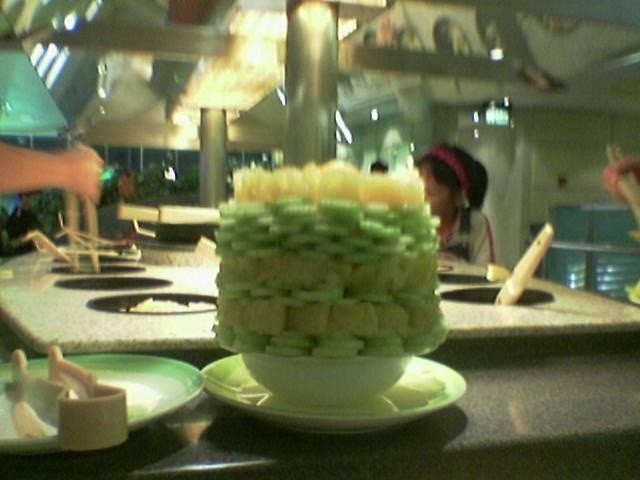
[212,196,447,360]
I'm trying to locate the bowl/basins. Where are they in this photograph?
[241,350,413,408]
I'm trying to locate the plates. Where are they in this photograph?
[200,354,467,435]
[0,353,206,457]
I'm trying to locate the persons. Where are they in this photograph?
[0,137,105,210]
[414,141,497,267]
[7,190,42,239]
